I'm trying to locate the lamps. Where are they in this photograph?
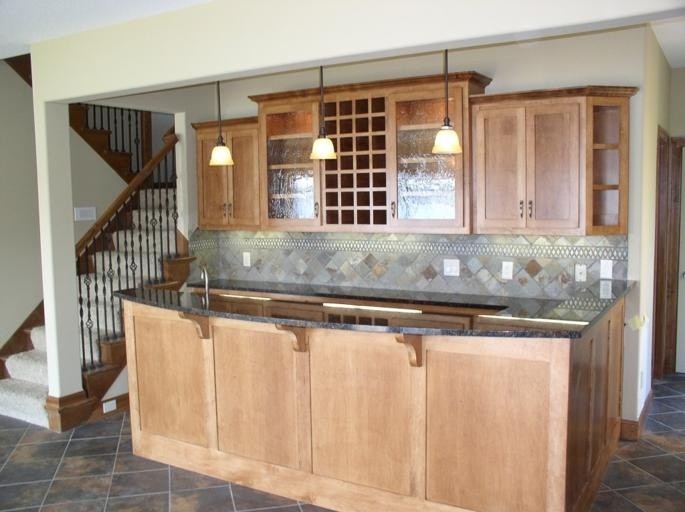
[307,64,339,162]
[431,47,465,156]
[206,79,238,168]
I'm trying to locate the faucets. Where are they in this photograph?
[199,265,210,313]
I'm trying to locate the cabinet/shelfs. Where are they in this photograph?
[471,100,586,234]
[262,100,322,229]
[196,127,260,229]
[387,84,485,235]
[320,95,390,233]
[586,96,629,233]
